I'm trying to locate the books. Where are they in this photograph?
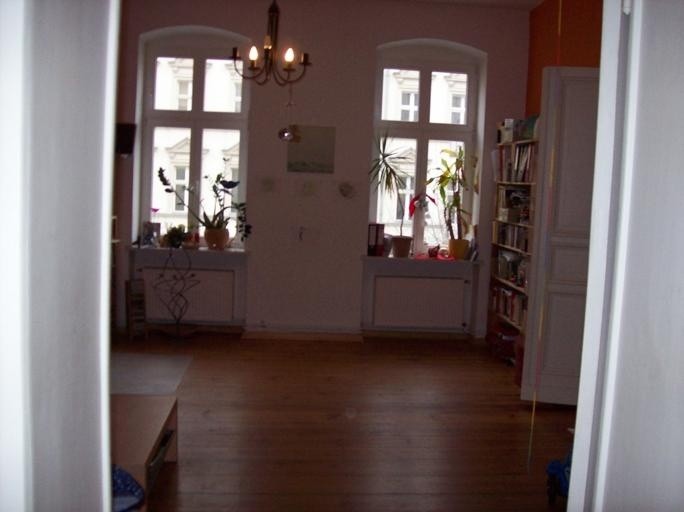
[491,144,538,330]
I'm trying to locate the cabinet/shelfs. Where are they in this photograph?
[486,139,538,362]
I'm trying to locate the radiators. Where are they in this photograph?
[138,268,235,323]
[373,275,467,328]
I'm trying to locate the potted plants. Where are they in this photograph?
[158,157,252,251]
[423,145,479,258]
[369,131,414,257]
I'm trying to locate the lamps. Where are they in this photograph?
[229,0,312,87]
[278,124,298,141]
